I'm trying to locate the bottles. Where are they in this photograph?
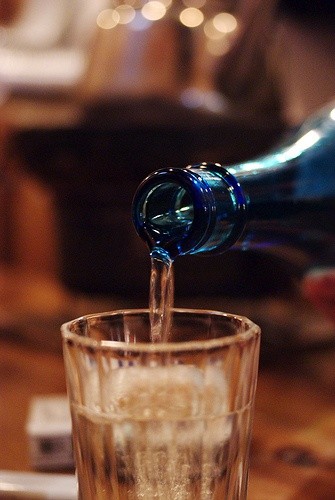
[134,98,335,262]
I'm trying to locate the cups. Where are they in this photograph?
[59,308,260,500]
